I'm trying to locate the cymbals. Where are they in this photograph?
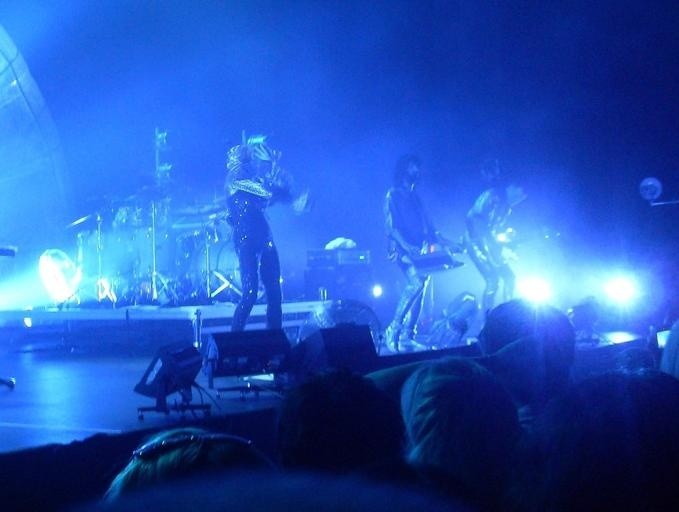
[170,202,222,217]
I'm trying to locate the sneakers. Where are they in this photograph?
[398,336,425,351]
[385,325,399,353]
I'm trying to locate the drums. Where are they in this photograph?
[177,228,220,260]
[193,235,266,301]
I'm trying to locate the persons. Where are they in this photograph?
[381,153,441,354]
[271,369,405,485]
[222,132,289,330]
[476,300,678,509]
[461,176,530,317]
[102,428,260,508]
